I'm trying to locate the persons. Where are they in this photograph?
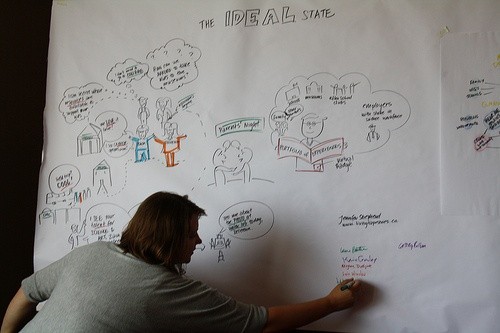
[0,191,363,333]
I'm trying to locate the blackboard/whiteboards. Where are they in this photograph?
[33,2,500,333]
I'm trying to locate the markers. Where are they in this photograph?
[341,280,355,292]
